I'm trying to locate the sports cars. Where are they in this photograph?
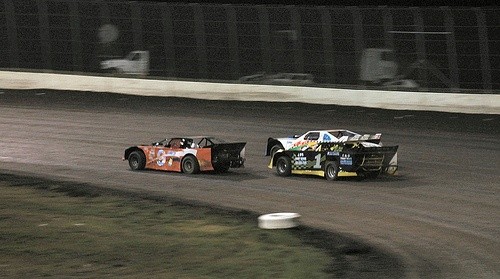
[265,129,383,159]
[123,136,248,175]
[268,140,398,181]
